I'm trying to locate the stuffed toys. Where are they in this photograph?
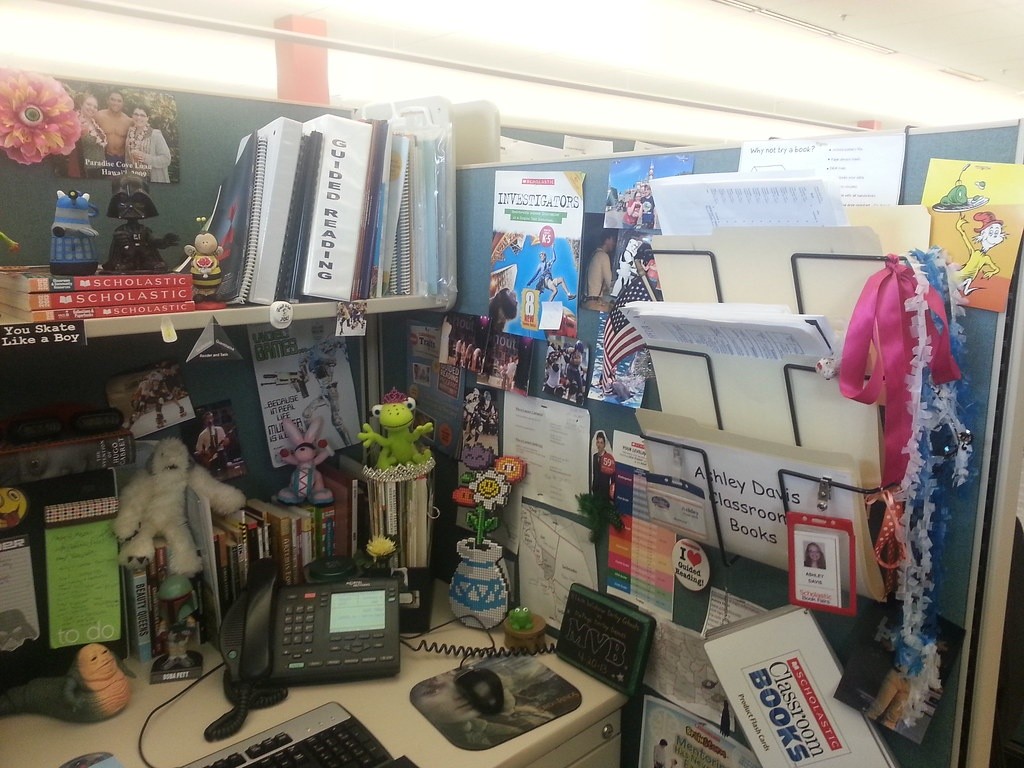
[357,389,433,472]
[114,440,242,578]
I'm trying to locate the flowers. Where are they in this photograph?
[451,443,527,545]
[360,534,400,569]
[0,68,81,165]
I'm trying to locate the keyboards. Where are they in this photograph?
[176,699,392,768]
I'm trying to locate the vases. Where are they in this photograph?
[449,537,510,631]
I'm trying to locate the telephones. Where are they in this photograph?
[218,558,399,685]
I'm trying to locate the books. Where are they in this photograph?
[0,113,457,669]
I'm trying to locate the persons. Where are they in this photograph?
[804,542,826,569]
[195,409,230,473]
[591,432,616,501]
[462,245,587,442]
[582,232,618,312]
[641,259,661,291]
[622,185,655,229]
[144,364,187,417]
[80,89,171,182]
[126,372,166,432]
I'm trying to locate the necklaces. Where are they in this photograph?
[129,122,150,150]
[76,110,108,148]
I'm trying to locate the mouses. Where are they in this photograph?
[454,666,505,715]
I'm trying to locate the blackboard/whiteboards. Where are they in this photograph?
[458,120,1024,768]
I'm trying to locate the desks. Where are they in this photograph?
[1,586,628,768]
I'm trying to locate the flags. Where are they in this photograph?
[599,276,658,395]
